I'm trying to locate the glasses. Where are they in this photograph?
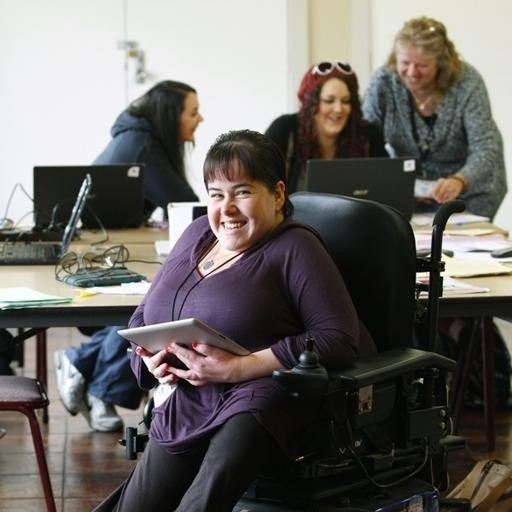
[311,62,353,75]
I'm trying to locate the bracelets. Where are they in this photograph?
[448,174,467,194]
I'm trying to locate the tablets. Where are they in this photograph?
[117,318,251,366]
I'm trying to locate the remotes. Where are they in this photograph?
[490,246,512,259]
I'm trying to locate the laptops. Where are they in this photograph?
[33,164,145,230]
[306,156,416,222]
[0,173,92,265]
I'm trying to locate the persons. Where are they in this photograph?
[53,80,205,437]
[112,127,377,511]
[364,16,509,225]
[265,60,381,195]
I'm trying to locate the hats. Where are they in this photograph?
[298,61,358,103]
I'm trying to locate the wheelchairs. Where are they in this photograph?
[116,190,464,511]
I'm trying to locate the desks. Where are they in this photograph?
[1,208,512,449]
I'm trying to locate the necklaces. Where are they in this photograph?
[408,87,439,112]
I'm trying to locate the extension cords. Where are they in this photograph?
[0,229,81,241]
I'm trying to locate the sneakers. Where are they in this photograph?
[54,350,87,416]
[84,389,124,432]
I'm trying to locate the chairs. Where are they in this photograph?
[228,189,472,512]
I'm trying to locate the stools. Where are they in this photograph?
[2,376,59,512]
